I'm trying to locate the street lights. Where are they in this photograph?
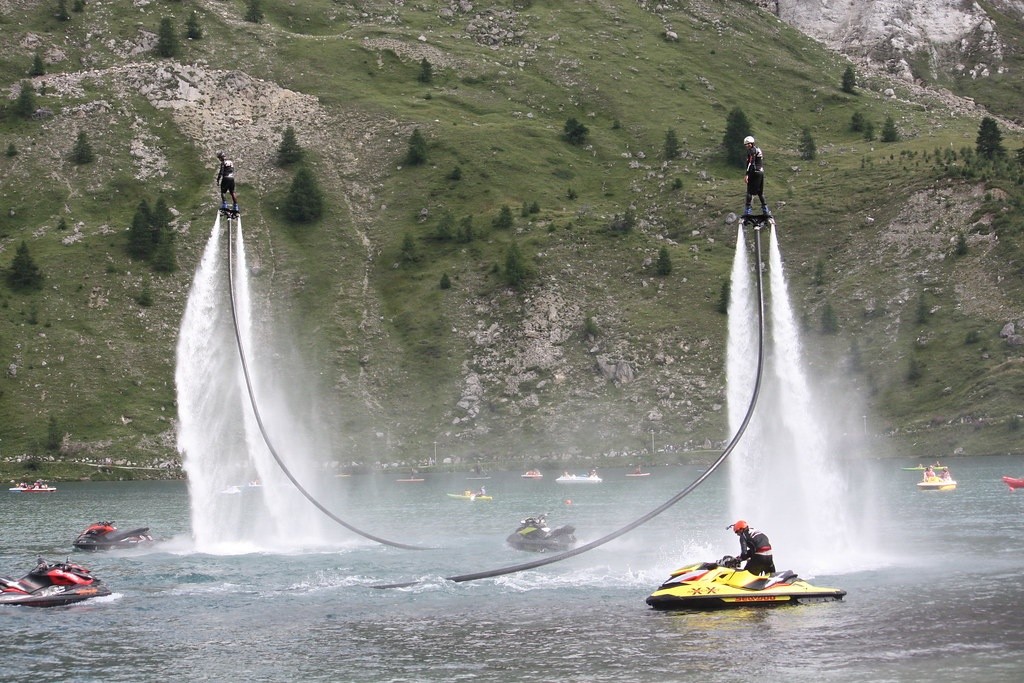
[650,429,654,452]
[433,442,437,464]
[861,415,867,433]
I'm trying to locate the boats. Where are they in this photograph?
[8,482,58,495]
[445,491,493,504]
[521,474,545,480]
[1002,476,1024,492]
[625,473,652,477]
[900,467,948,471]
[396,478,426,483]
[555,476,604,484]
[249,481,264,489]
[919,476,957,491]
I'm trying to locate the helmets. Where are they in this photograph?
[744,136,755,146]
[726,521,749,534]
[218,152,226,159]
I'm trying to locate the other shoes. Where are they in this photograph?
[231,204,239,210]
[745,207,752,214]
[221,201,228,208]
[761,206,769,213]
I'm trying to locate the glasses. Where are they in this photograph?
[745,143,750,145]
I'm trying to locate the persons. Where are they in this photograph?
[30,558,54,574]
[216,152,239,211]
[405,464,648,503]
[743,135,770,215]
[916,457,952,485]
[515,518,546,539]
[733,519,775,576]
[10,478,51,491]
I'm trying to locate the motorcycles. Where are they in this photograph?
[508,519,579,553]
[0,560,114,604]
[75,521,155,552]
[645,556,847,609]
[222,486,240,495]
